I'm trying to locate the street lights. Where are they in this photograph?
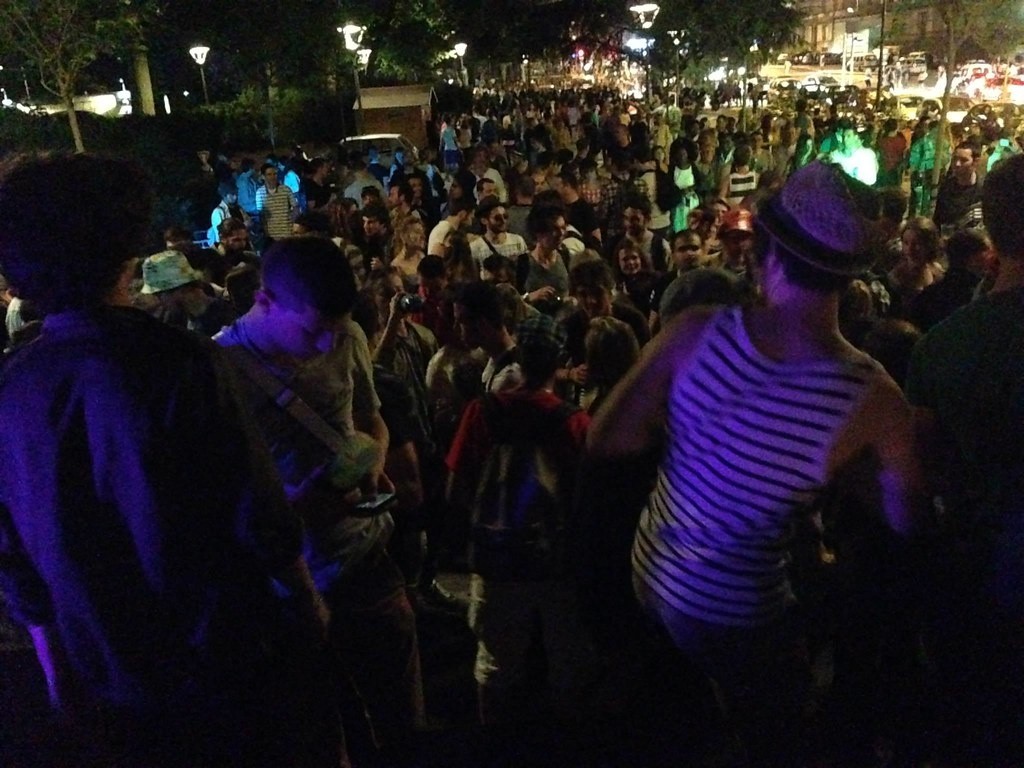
[337,17,372,135]
[188,38,211,103]
[628,0,660,132]
[454,39,469,69]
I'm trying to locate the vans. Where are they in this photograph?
[953,60,992,83]
[852,53,877,72]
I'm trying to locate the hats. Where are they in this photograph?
[478,194,507,216]
[524,123,552,148]
[752,160,882,275]
[218,182,240,196]
[518,315,566,354]
[141,250,203,295]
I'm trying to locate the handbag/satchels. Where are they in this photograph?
[323,433,379,487]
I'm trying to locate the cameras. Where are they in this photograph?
[351,488,400,515]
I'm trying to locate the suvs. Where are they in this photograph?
[339,133,419,162]
[899,58,929,80]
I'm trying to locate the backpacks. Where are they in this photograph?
[470,393,552,571]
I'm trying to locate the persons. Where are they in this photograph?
[0,87,1024,709]
[645,229,704,339]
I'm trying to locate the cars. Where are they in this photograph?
[979,77,1024,104]
[881,94,923,120]
[793,51,842,65]
[954,73,998,98]
[917,96,978,125]
[847,87,895,113]
[769,75,801,102]
[800,75,846,94]
[960,101,1024,134]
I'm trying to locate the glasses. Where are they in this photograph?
[494,215,509,221]
